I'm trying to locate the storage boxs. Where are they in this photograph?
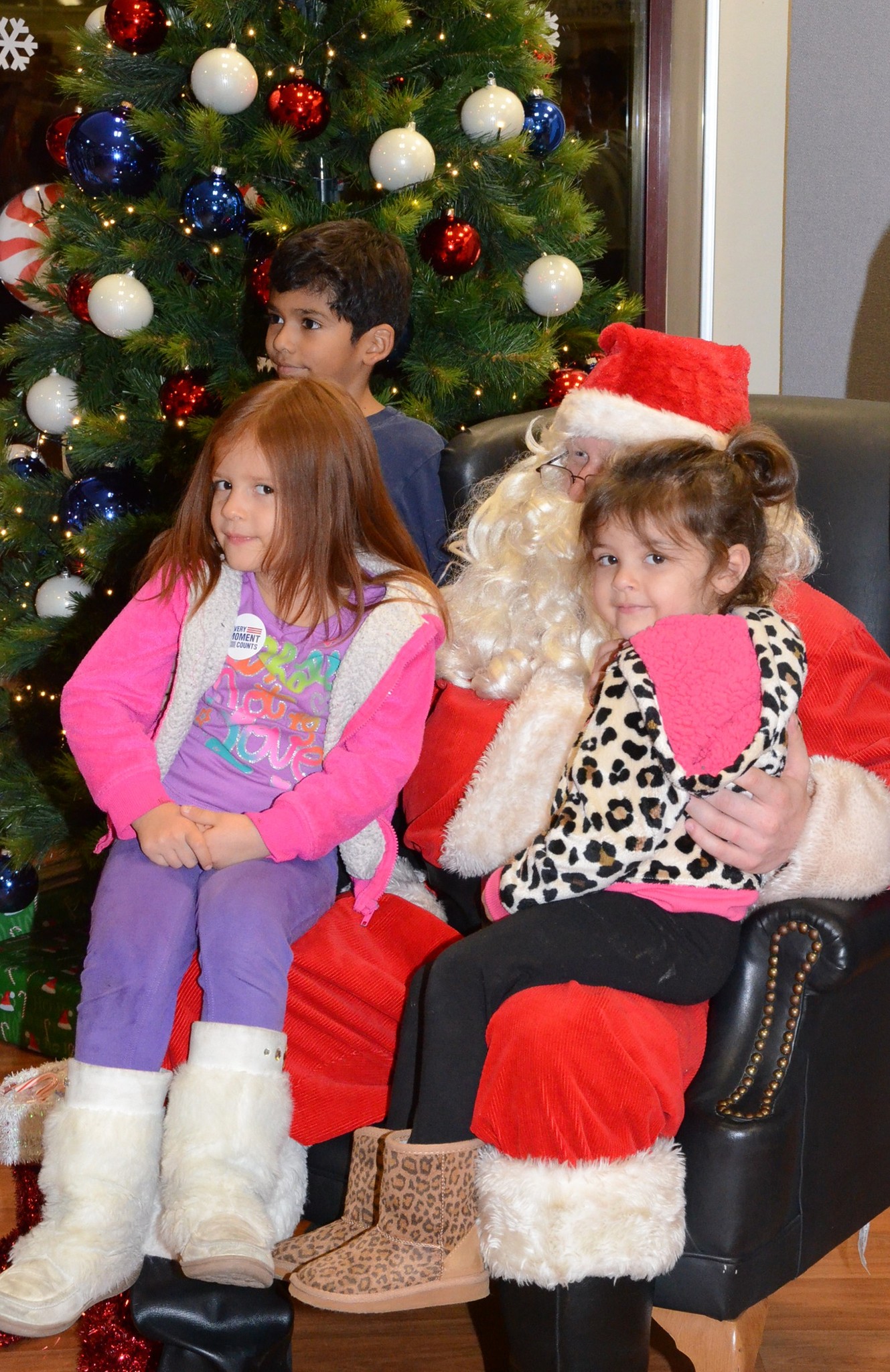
[1,914,95,1061]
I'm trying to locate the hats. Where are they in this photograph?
[553,322,751,453]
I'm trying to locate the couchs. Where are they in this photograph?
[295,396,890,1371]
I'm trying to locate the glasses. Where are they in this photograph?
[535,451,605,496]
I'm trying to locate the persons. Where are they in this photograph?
[262,426,799,1313]
[250,219,471,607]
[123,321,889,1372]
[0,378,451,1333]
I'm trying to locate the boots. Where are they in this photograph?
[128,1133,294,1372]
[1,1057,172,1337]
[271,1125,397,1283]
[474,1136,687,1372]
[289,1126,491,1313]
[156,1019,292,1289]
